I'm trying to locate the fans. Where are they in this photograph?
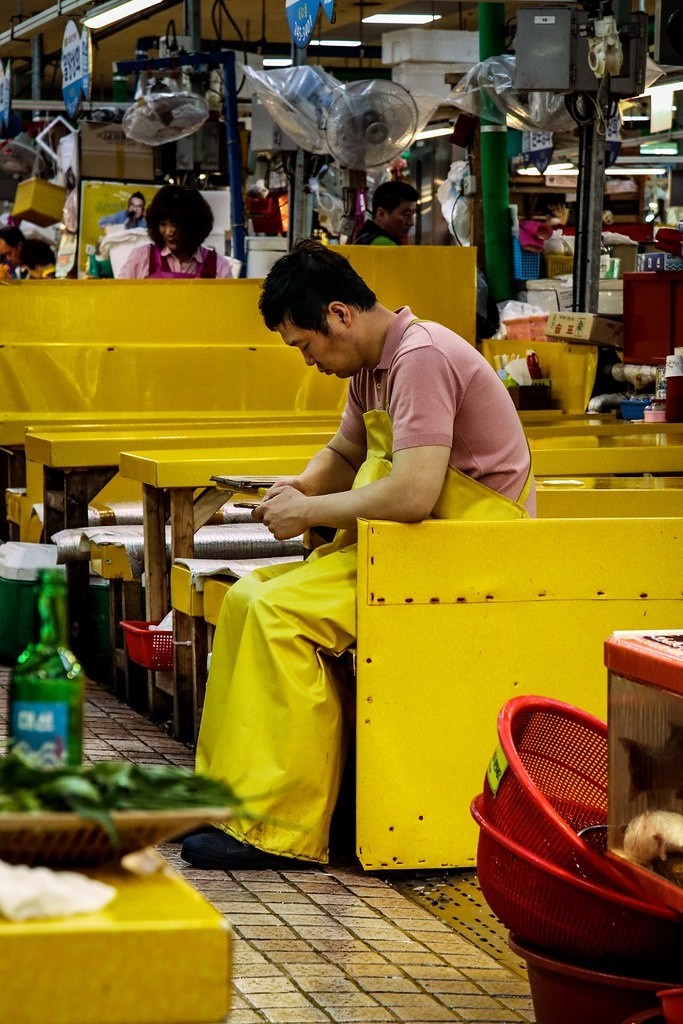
[259,66,334,152]
[324,79,418,171]
[122,68,208,147]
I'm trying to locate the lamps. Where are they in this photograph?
[81,0,163,30]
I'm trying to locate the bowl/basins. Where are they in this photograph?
[644,411,666,423]
[619,400,651,419]
[665,355,683,379]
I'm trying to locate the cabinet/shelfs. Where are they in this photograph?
[622,272,683,362]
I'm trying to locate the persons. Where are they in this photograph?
[99,191,146,229]
[117,186,233,279]
[167,239,537,870]
[351,180,421,245]
[0,226,56,280]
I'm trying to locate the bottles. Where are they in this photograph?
[6,567,85,774]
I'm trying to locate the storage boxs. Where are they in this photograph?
[543,254,573,278]
[392,62,475,97]
[605,243,638,272]
[514,236,539,280]
[543,312,621,347]
[501,315,563,342]
[382,28,479,65]
[605,630,683,913]
[11,178,66,228]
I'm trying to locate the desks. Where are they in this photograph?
[0,411,683,742]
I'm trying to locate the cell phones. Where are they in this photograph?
[234,503,260,508]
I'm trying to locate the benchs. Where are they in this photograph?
[28,502,261,525]
[80,526,302,711]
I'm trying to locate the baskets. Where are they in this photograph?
[544,255,573,279]
[0,808,241,869]
[514,238,540,280]
[119,621,174,670]
[470,696,683,966]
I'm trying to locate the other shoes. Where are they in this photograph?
[165,823,318,870]
